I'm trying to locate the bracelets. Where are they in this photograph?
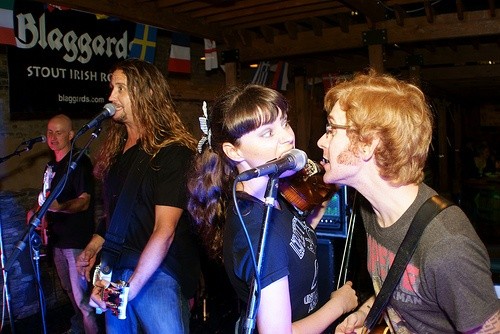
[361,304,371,310]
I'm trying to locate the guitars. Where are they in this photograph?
[26,165,56,247]
[88,258,129,320]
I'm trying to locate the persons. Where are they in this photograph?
[317,66,500,334]
[74,58,199,334]
[26,113,106,334]
[461,141,497,216]
[185,83,359,334]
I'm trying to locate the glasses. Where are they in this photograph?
[325,123,349,137]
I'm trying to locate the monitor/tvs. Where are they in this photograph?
[315,182,348,239]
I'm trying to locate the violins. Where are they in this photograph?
[277,157,340,211]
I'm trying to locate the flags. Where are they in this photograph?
[0,1,288,91]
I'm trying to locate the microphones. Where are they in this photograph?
[237,148,308,183]
[77,102,116,137]
[21,135,46,146]
